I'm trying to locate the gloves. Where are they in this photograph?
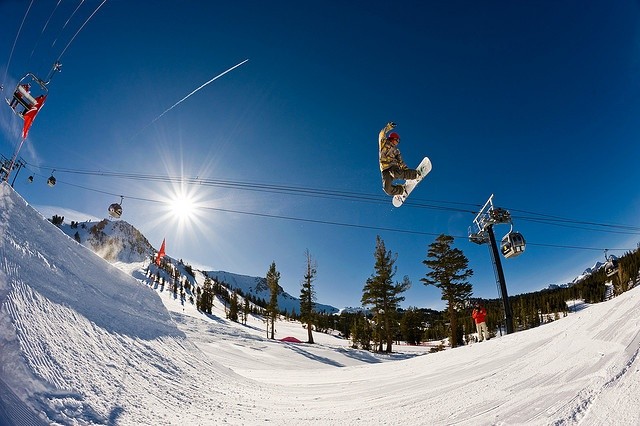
[390,122,397,127]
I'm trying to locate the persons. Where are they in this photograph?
[21,95,43,115]
[9,83,32,109]
[472,302,490,343]
[379,122,421,198]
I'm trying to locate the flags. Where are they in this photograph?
[24,95,46,138]
[156,239,165,266]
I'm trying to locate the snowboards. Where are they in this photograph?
[392,157,433,207]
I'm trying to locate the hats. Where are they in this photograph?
[389,132,398,139]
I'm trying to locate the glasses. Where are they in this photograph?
[395,138,400,143]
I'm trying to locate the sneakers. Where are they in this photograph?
[402,186,408,198]
[416,170,423,183]
